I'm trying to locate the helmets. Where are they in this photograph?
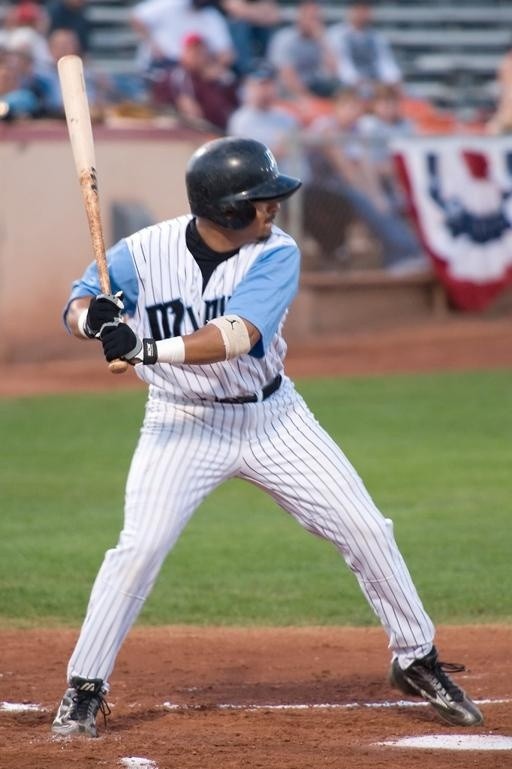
[184,135,304,231]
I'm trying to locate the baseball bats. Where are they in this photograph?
[57,55,126,374]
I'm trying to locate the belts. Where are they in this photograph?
[200,374,283,404]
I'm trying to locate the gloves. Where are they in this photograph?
[83,289,159,367]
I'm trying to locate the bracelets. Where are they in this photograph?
[76,308,89,340]
[154,334,186,366]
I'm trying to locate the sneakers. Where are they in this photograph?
[50,676,110,740]
[386,645,484,728]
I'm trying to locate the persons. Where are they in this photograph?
[129,0,512,270]
[46,138,483,741]
[1,1,107,123]
